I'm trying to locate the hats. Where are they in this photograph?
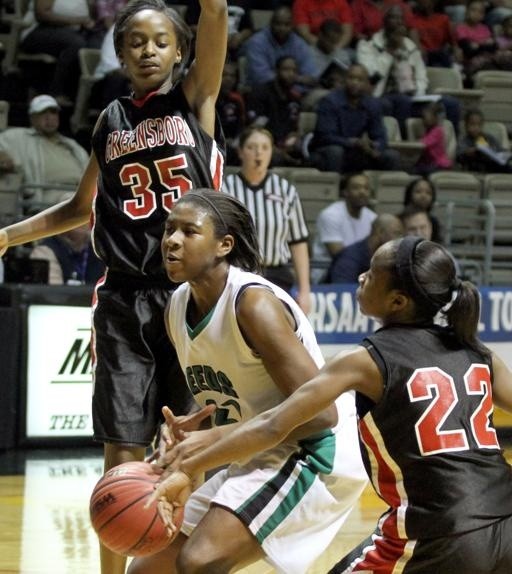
[27,93,62,116]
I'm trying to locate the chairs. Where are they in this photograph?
[298,109,319,138]
[1,0,55,76]
[223,163,511,287]
[0,169,80,252]
[381,67,512,163]
[69,47,104,133]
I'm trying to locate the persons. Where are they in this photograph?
[125,190,368,574]
[144,238,512,574]
[0,0,232,574]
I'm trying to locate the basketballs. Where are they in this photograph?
[90,461,184,555]
